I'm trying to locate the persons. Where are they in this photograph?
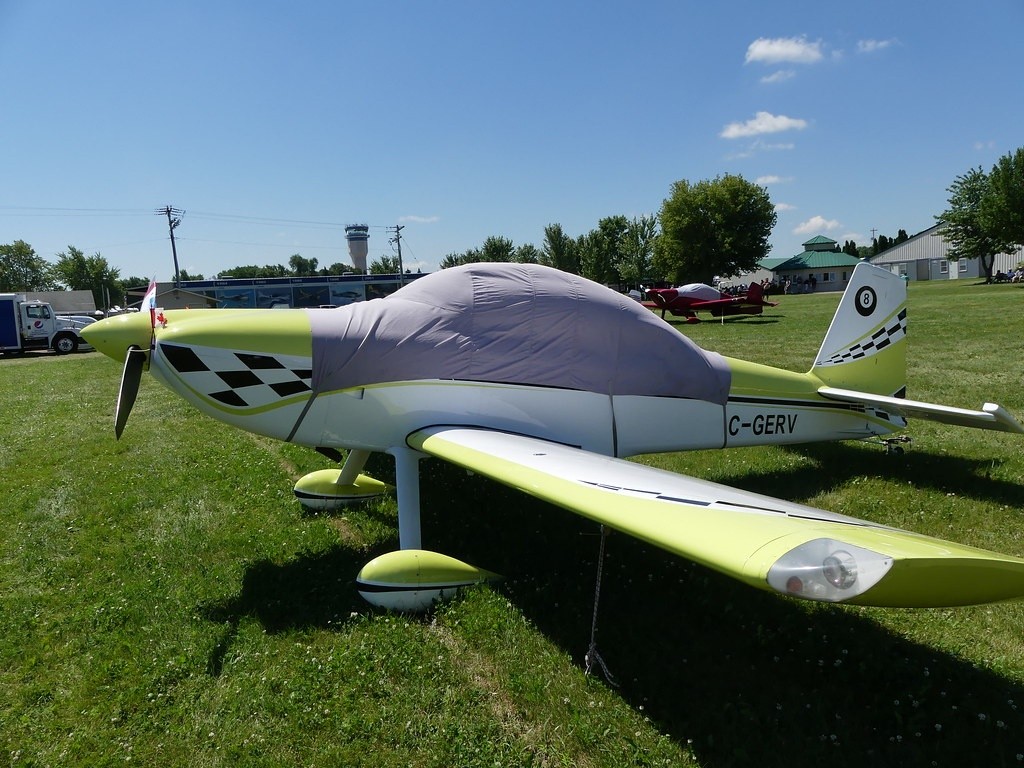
[761,278,771,301]
[787,274,817,294]
[988,267,1024,284]
[722,285,743,296]
[901,273,909,286]
[645,286,650,292]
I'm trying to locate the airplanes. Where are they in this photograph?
[640,282,779,324]
[80,263,1024,612]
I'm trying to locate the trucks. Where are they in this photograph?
[0,293,98,355]
